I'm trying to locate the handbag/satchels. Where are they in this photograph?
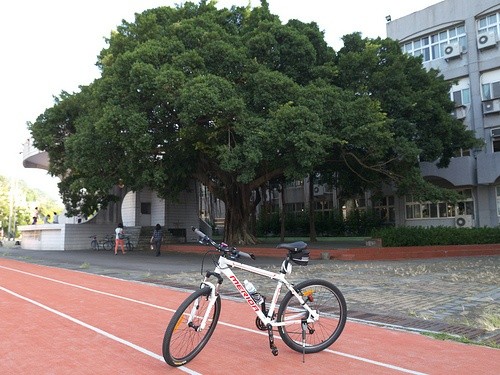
[150,245,154,250]
[118,233,125,239]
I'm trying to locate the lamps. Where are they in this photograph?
[386,15,391,22]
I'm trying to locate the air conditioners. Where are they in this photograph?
[476,32,496,49]
[444,44,460,60]
[313,185,323,196]
[482,100,500,114]
[449,107,466,119]
[455,215,472,229]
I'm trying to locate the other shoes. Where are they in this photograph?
[116,253,118,255]
[123,252,127,254]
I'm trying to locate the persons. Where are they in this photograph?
[32,207,39,225]
[150,224,164,257]
[53,212,59,224]
[115,223,127,255]
[44,215,51,224]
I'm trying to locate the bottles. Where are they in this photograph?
[244,280,264,305]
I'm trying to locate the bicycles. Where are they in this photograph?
[88,234,101,252]
[162,227,349,367]
[103,233,119,253]
[124,235,135,253]
